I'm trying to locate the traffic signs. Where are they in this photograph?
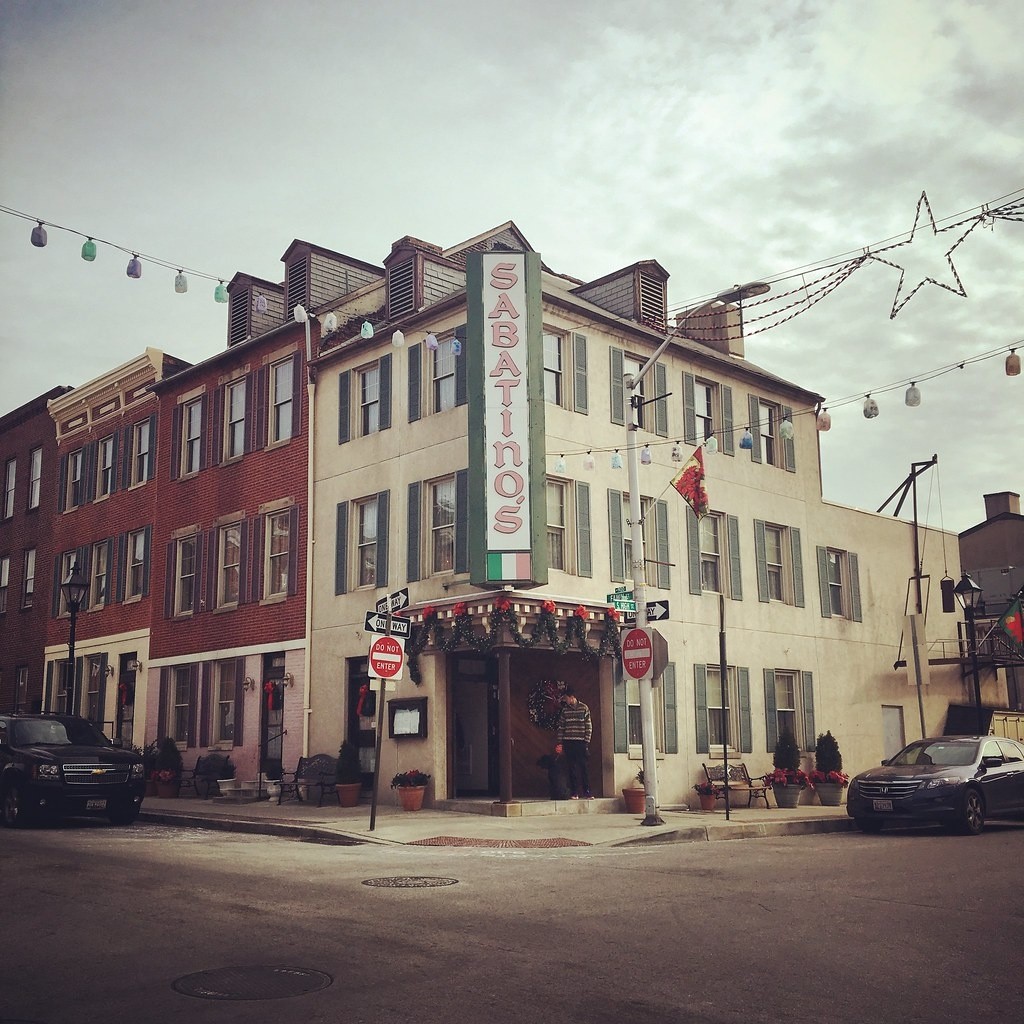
[364,610,411,640]
[375,586,411,616]
[623,600,670,624]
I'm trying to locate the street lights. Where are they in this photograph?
[952,568,985,736]
[621,280,772,826]
[59,561,91,716]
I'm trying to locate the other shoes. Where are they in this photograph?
[570,792,579,799]
[586,794,594,800]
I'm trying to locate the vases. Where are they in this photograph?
[397,786,424,811]
[701,794,714,811]
[773,784,802,808]
[622,788,647,814]
[814,782,843,806]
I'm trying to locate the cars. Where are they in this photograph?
[845,734,1024,836]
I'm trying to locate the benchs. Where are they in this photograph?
[273,754,344,807]
[701,762,770,808]
[177,755,229,800]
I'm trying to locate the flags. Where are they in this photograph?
[669,444,710,523]
[998,597,1024,652]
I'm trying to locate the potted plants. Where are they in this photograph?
[130,738,180,798]
[217,763,236,796]
[334,741,363,807]
[263,758,282,802]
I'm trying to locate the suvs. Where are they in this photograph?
[0,710,146,829]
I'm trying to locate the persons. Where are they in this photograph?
[557,687,595,800]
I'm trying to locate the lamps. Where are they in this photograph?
[105,664,114,678]
[243,676,256,692]
[283,672,294,687]
[132,659,142,673]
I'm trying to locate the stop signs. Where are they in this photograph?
[621,626,654,681]
[367,634,405,680]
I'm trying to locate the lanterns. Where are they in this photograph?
[553,354,1021,471]
[31,224,461,355]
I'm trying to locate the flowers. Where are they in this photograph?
[694,782,719,795]
[391,770,432,788]
[763,768,814,791]
[810,770,849,788]
